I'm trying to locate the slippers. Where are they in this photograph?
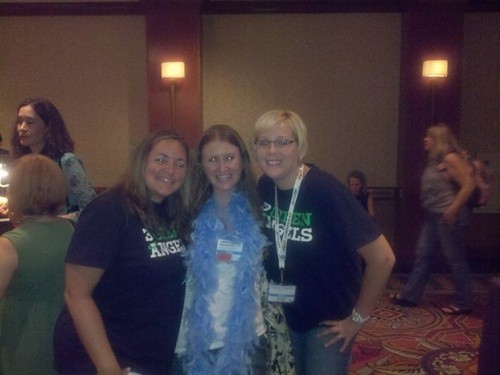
[389,293,416,307]
[440,302,472,315]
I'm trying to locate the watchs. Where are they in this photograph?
[352,307,371,324]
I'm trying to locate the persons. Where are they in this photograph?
[253,110,396,375]
[0,154,76,375]
[9,97,97,222]
[388,123,475,314]
[173,124,269,375]
[54,129,192,375]
[347,169,374,215]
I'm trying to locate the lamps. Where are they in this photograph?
[422,60,449,125]
[162,62,186,134]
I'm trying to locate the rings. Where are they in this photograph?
[338,334,342,339]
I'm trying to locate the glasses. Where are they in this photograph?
[255,138,296,149]
